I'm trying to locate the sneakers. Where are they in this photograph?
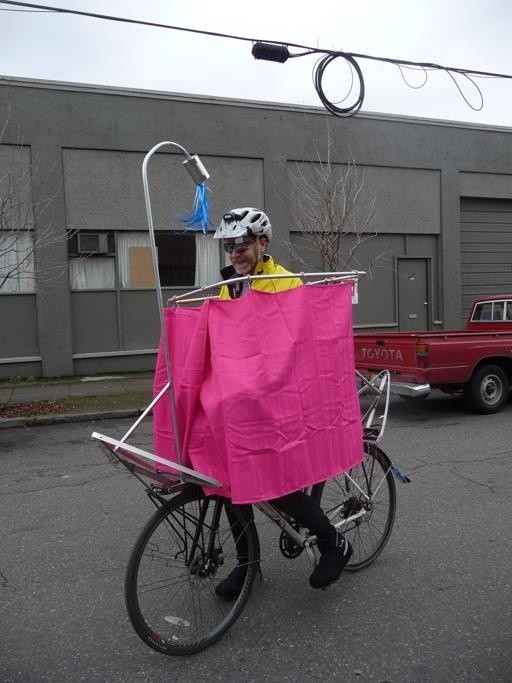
[214,559,263,601]
[309,534,353,588]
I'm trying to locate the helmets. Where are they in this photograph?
[213,207,272,242]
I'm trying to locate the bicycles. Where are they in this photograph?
[122,420,409,658]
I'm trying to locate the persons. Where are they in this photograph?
[213,207,354,598]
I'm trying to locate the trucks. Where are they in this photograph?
[355,293,512,416]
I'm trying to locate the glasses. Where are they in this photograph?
[222,240,256,253]
